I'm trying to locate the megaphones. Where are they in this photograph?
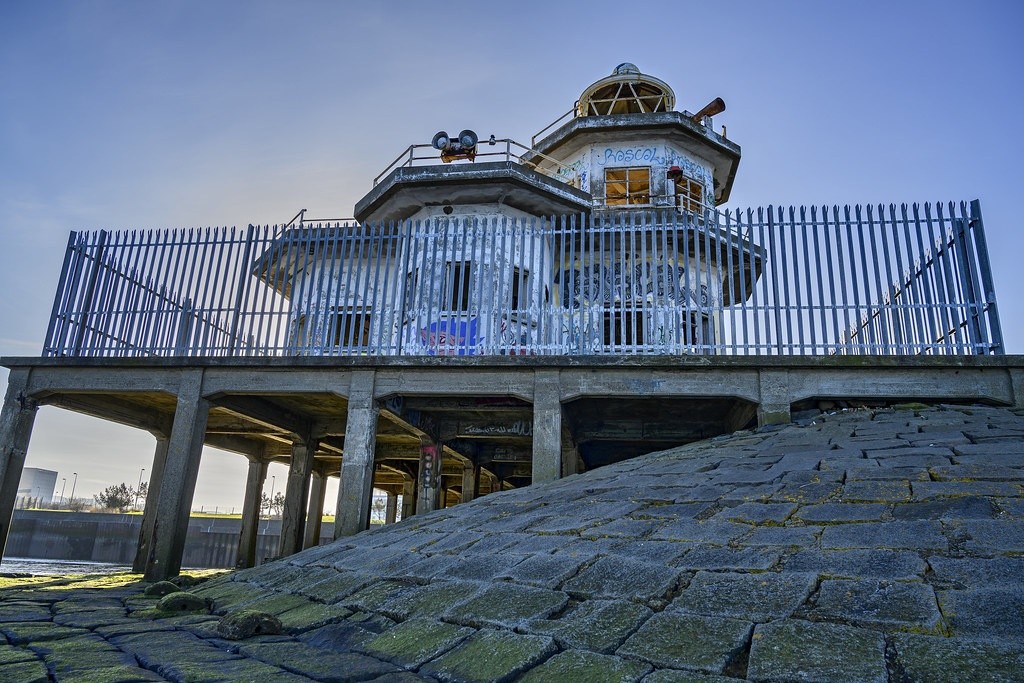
[459,129,478,149]
[431,130,452,151]
